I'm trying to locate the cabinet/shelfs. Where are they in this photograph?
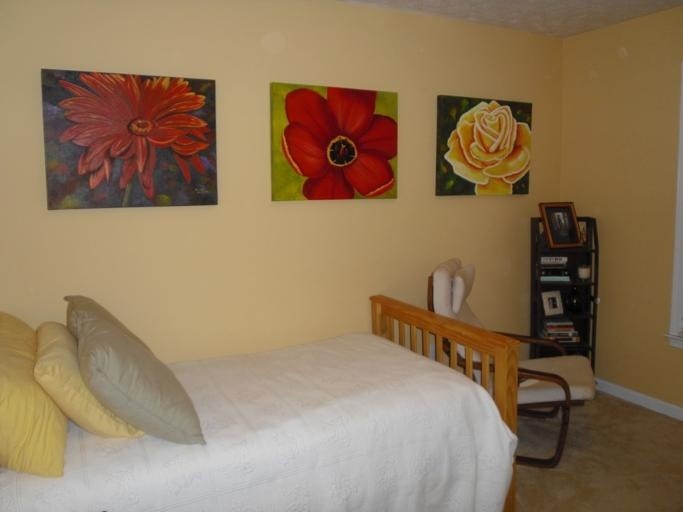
[530,217,598,375]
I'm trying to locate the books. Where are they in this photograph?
[539,255,570,283]
[540,320,581,345]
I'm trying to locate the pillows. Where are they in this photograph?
[1,295,206,480]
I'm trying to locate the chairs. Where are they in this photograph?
[425,256,596,469]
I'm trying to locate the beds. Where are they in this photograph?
[1,294,519,512]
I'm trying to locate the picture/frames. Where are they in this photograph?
[539,201,584,249]
